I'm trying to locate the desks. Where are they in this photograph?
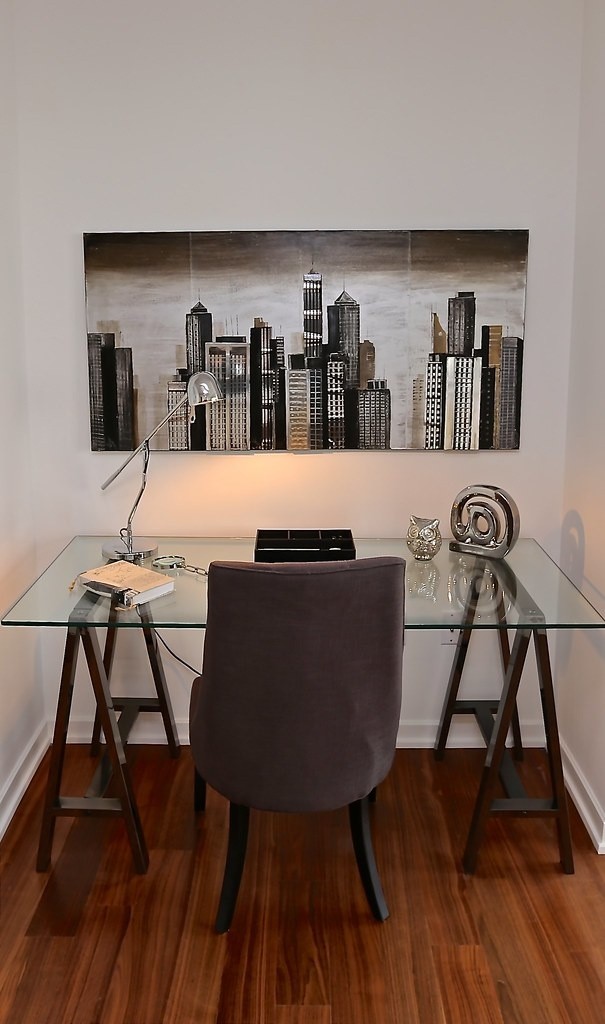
[1,536,605,874]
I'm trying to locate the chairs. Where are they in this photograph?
[189,556,407,934]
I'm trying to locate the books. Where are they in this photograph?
[80,560,176,608]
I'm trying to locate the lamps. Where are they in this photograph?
[99,370,225,565]
